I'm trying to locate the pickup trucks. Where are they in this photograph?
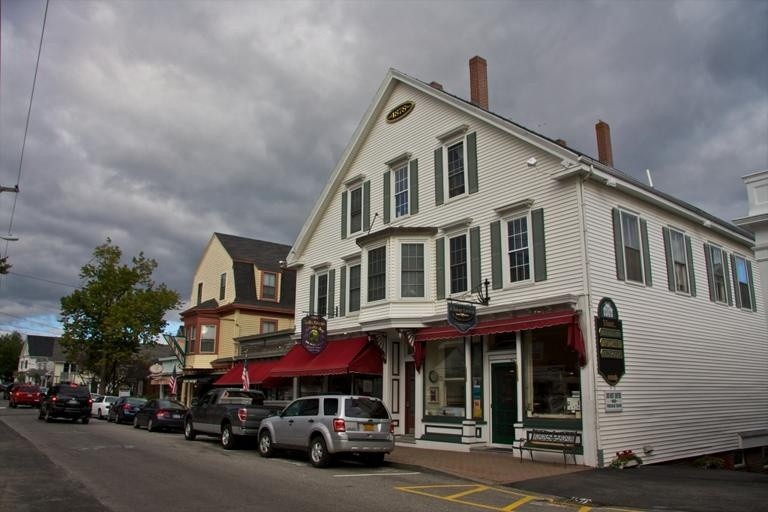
[182,386,285,450]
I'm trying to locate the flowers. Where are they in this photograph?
[613,451,634,461]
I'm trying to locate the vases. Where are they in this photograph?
[617,460,639,470]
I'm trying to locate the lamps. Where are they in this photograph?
[525,155,570,176]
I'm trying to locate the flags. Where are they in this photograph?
[167,365,178,395]
[241,355,250,391]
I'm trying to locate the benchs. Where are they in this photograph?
[517,429,579,469]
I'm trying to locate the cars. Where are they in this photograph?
[0,378,121,417]
[103,396,148,426]
[131,397,190,432]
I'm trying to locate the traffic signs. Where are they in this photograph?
[598,337,623,349]
[597,327,622,339]
[600,347,624,359]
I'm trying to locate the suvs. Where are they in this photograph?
[256,393,398,468]
[35,380,94,425]
